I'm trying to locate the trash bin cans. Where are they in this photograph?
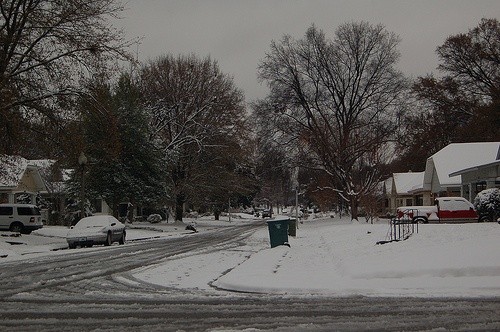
[266,220,288,248]
[288,219,296,236]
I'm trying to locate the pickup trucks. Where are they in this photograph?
[397,197,479,223]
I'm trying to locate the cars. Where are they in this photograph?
[66,216,126,249]
[262,209,272,218]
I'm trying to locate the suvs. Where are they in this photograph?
[0,203,43,235]
